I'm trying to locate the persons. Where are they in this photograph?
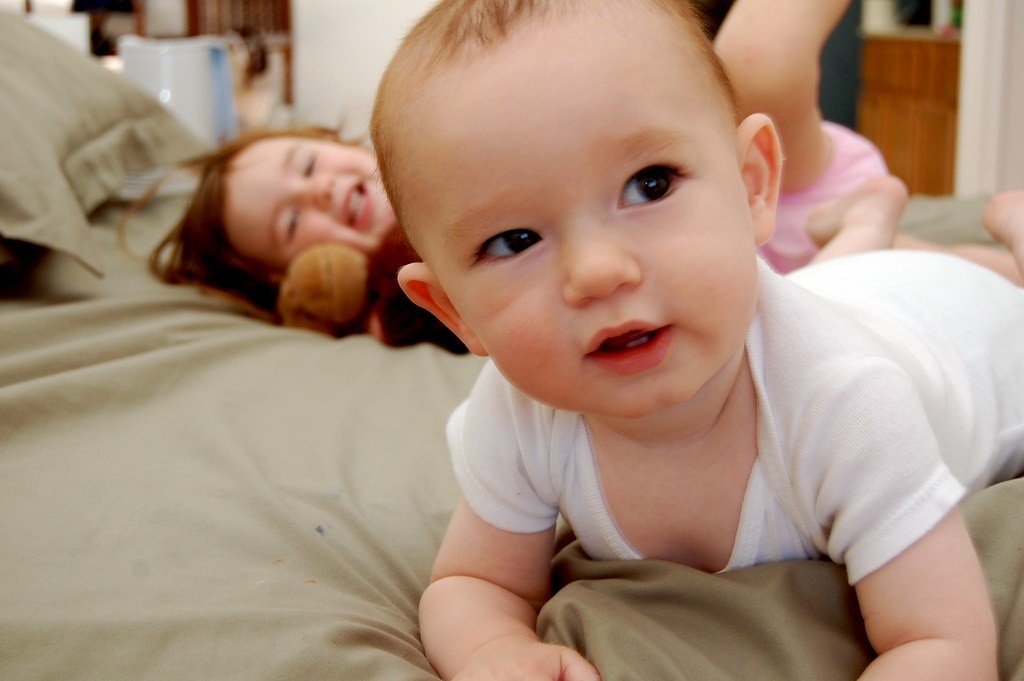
[368,0,1023,681]
[150,0,1023,353]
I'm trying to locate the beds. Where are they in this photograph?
[0,191,1024,681]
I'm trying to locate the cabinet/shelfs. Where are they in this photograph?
[855,32,960,198]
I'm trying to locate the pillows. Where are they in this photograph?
[0,8,204,284]
